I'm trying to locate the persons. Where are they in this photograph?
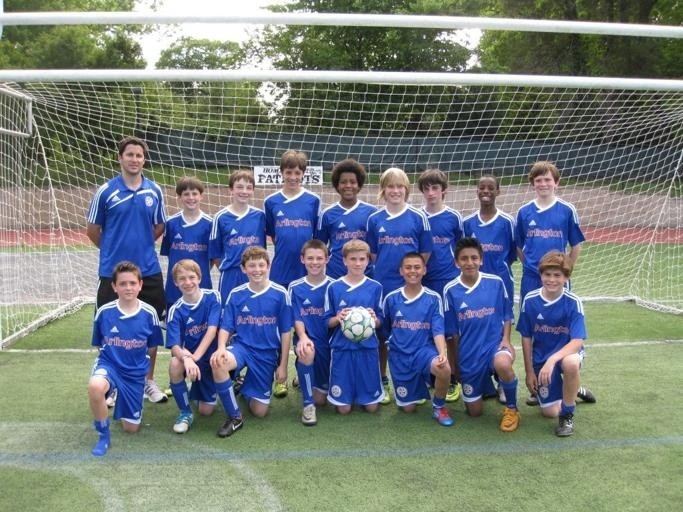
[382,251,455,428]
[166,258,222,435]
[85,260,164,456]
[367,168,433,406]
[463,174,517,404]
[442,238,521,432]
[514,162,585,406]
[316,158,378,279]
[287,240,337,426]
[324,239,384,415]
[211,172,267,393]
[516,249,596,437]
[87,136,168,402]
[160,177,214,397]
[263,149,323,397]
[415,169,463,403]
[209,247,291,438]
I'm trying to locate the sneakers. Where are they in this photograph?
[498,387,506,403]
[173,413,193,433]
[526,394,539,405]
[274,382,287,395]
[106,388,117,406]
[165,385,173,395]
[577,387,596,403]
[293,376,299,386]
[143,380,168,402]
[445,383,460,402]
[558,413,574,436]
[234,377,244,395]
[379,385,390,403]
[417,399,427,405]
[302,406,316,424]
[218,418,243,437]
[501,408,519,432]
[432,403,453,426]
[92,432,110,455]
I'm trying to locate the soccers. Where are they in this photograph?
[340,306,376,342]
